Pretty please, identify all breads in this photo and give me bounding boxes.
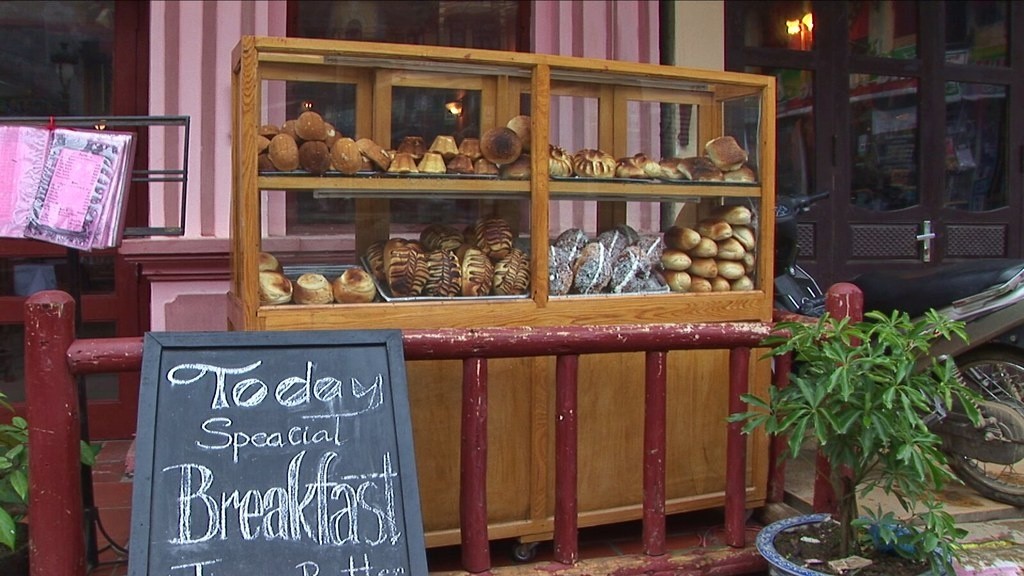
[548,136,756,183]
[257,111,531,173]
[258,214,532,304]
[548,204,756,294]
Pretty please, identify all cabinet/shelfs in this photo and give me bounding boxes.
[227,34,777,566]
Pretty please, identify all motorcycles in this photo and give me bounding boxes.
[750,189,1024,512]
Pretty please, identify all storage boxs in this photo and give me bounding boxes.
[746,17,1008,119]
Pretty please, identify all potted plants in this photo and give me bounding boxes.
[0,390,104,576]
[721,308,984,576]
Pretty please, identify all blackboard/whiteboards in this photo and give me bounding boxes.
[128,328,431,576]
